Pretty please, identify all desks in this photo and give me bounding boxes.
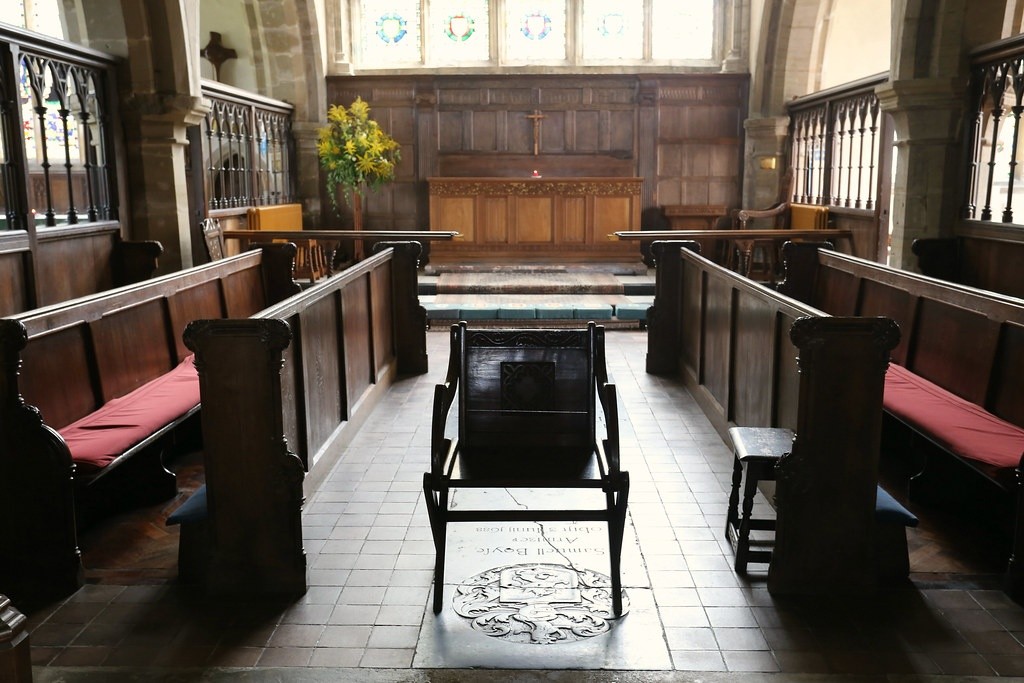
[426,176,646,264]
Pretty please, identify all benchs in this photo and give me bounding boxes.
[772,234,1024,607]
[0,238,303,595]
[643,236,921,608]
[159,239,431,606]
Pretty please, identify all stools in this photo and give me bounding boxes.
[723,418,802,578]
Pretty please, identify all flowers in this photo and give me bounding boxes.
[311,90,406,217]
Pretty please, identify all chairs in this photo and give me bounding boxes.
[418,308,634,626]
[159,310,312,615]
